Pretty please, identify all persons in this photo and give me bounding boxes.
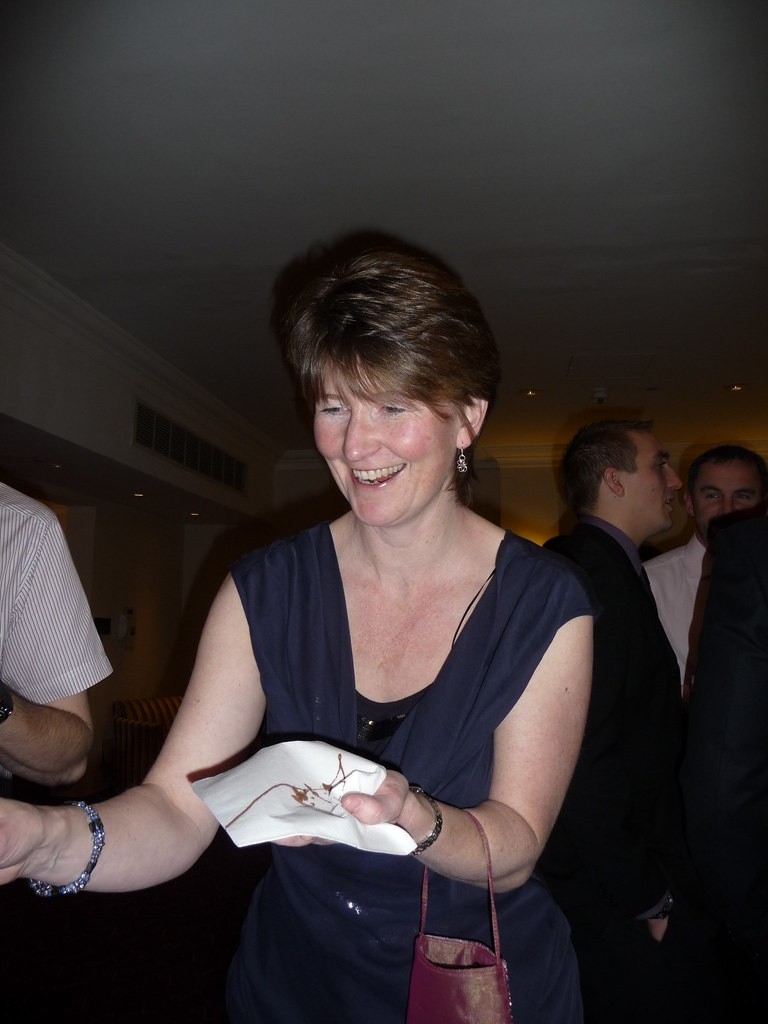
[685,496,765,1024]
[0,479,113,1019]
[638,442,765,700]
[0,245,599,1024]
[541,416,690,1022]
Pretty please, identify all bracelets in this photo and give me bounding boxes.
[650,891,674,920]
[0,680,14,725]
[28,798,107,899]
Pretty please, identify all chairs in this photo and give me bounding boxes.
[112,695,184,792]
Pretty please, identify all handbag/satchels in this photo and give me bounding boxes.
[406,804,515,1024]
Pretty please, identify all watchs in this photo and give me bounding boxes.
[399,784,444,861]
